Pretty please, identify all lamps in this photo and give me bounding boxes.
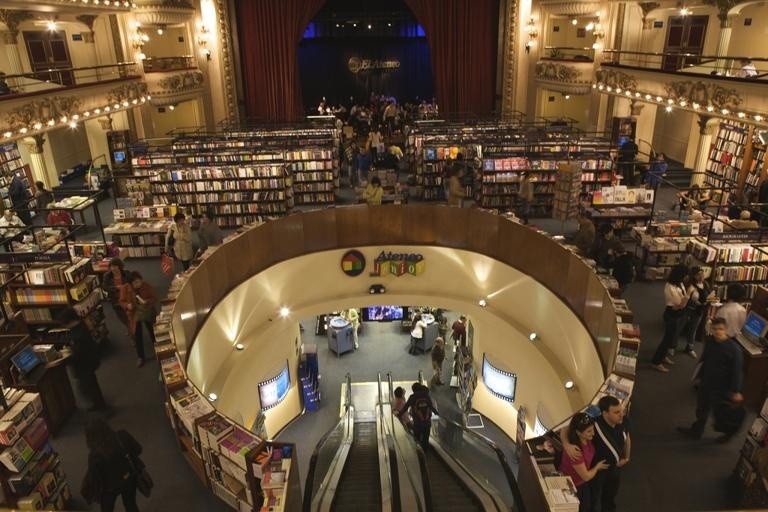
[127,20,149,64]
[196,23,216,62]
[526,12,540,55]
[590,10,607,51]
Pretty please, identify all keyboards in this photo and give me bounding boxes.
[736,335,762,355]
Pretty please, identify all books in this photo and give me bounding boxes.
[0,125,765,511]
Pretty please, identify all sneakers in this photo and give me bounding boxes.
[137,358,142,367]
[651,349,696,372]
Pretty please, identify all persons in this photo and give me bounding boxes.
[318,89,440,124]
[730,55,758,80]
[0,72,14,94]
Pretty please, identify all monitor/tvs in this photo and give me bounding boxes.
[742,310,768,338]
[11,344,40,376]
[360,306,409,322]
[618,137,629,145]
[114,152,125,161]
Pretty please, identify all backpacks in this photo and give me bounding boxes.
[414,395,431,421]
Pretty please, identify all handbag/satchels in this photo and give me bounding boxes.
[714,401,744,432]
[168,230,174,245]
[134,305,153,322]
[162,254,173,273]
[136,469,153,497]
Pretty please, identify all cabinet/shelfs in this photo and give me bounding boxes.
[327,317,358,356]
[116,123,338,260]
[154,346,296,511]
[410,320,440,356]
[361,118,518,235]
